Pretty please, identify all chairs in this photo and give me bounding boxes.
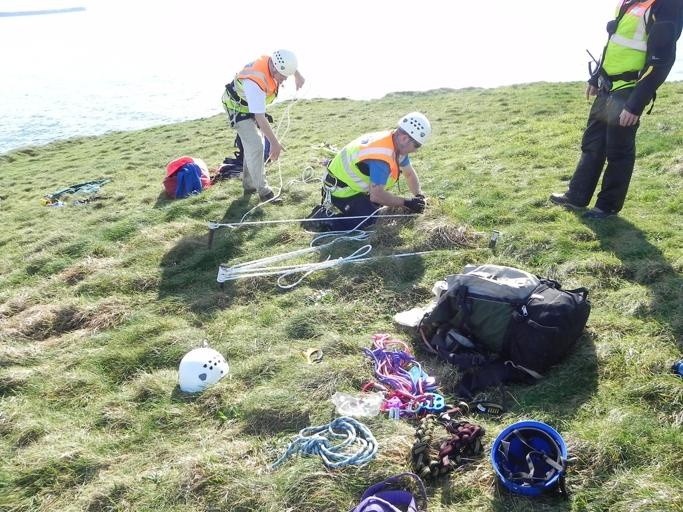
[301,205,331,230]
[243,188,283,205]
[549,192,618,219]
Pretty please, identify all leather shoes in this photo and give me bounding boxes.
[405,196,426,214]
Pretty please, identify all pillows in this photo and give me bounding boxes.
[491,420,568,495]
[178,346,228,393]
[270,49,297,78]
[398,112,430,145]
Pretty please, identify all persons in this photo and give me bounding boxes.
[300,111,433,233]
[222,50,303,203]
[548,0,683,223]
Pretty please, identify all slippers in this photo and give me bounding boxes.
[162,156,211,199]
[420,264,589,381]
